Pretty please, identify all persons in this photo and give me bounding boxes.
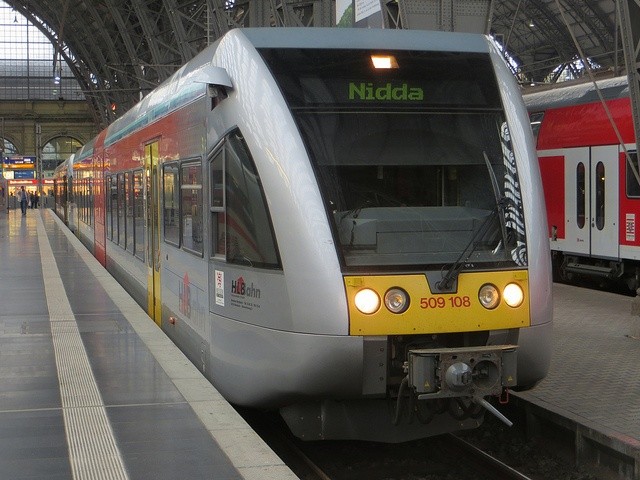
[18,186,28,216]
[28,190,39,209]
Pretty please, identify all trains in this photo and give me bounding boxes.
[517,72,640,296]
[53,26,555,445]
